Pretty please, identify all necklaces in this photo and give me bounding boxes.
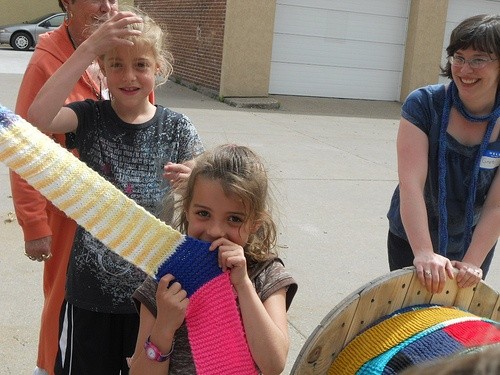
[71,37,94,64]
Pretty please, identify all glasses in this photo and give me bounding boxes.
[447,54,498,69]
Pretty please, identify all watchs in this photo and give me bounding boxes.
[144,335,175,362]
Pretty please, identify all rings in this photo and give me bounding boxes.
[24,253,50,261]
[475,270,480,274]
[425,271,431,276]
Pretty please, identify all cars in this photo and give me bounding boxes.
[0,12,67,50]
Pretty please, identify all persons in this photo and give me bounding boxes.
[386,14,500,294]
[9,0,154,375]
[129,144,298,375]
[26,5,206,375]
